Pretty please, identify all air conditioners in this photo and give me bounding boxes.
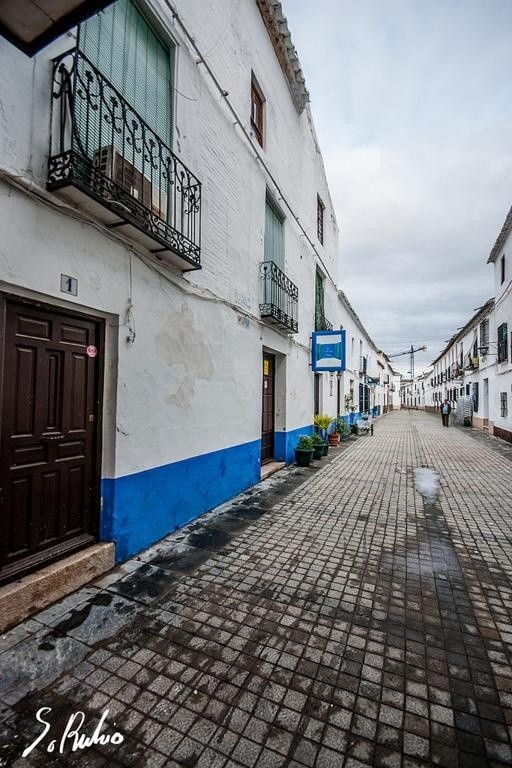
[88,145,152,221]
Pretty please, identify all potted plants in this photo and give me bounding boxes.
[293,414,353,466]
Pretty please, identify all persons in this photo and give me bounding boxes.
[439,399,451,427]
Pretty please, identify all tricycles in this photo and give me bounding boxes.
[353,410,373,436]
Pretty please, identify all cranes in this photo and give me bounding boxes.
[386,344,428,378]
